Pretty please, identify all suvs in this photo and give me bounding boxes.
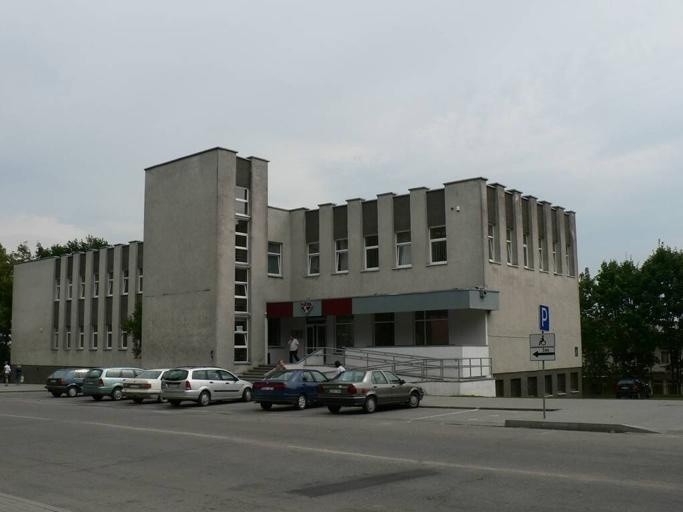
[158,365,254,408]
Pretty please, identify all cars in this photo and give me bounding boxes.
[250,368,330,412]
[120,367,172,404]
[44,366,89,398]
[615,378,651,399]
[317,367,425,414]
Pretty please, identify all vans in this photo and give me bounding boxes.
[79,367,146,401]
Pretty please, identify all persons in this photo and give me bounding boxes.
[13,363,22,385]
[1,360,12,387]
[335,360,346,376]
[276,360,287,371]
[287,334,300,363]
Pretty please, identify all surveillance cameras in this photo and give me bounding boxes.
[456,205,460,212]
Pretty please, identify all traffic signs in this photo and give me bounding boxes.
[528,332,556,362]
